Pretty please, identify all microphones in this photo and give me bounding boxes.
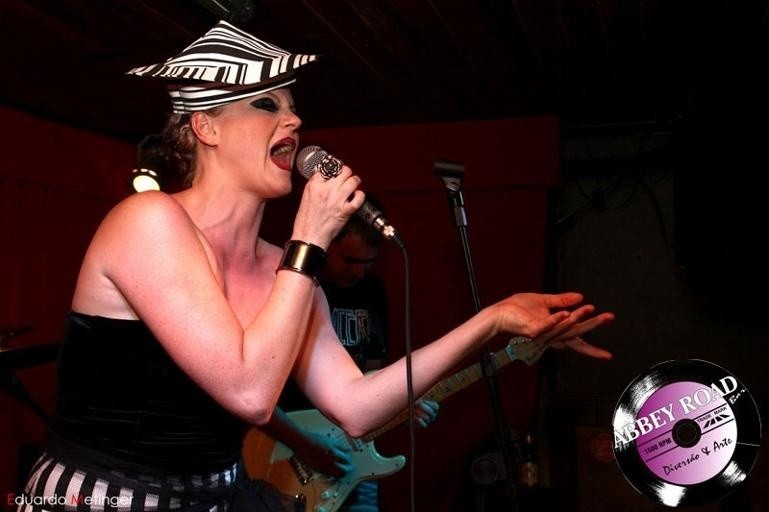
[296,145,400,241]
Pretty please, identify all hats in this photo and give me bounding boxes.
[125,19,318,115]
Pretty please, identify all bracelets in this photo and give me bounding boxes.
[276,240,327,288]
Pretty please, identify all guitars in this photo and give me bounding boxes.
[242,332,548,511]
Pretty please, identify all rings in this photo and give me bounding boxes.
[317,155,343,179]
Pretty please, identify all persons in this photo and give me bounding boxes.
[14,19,614,512]
[239,192,440,512]
